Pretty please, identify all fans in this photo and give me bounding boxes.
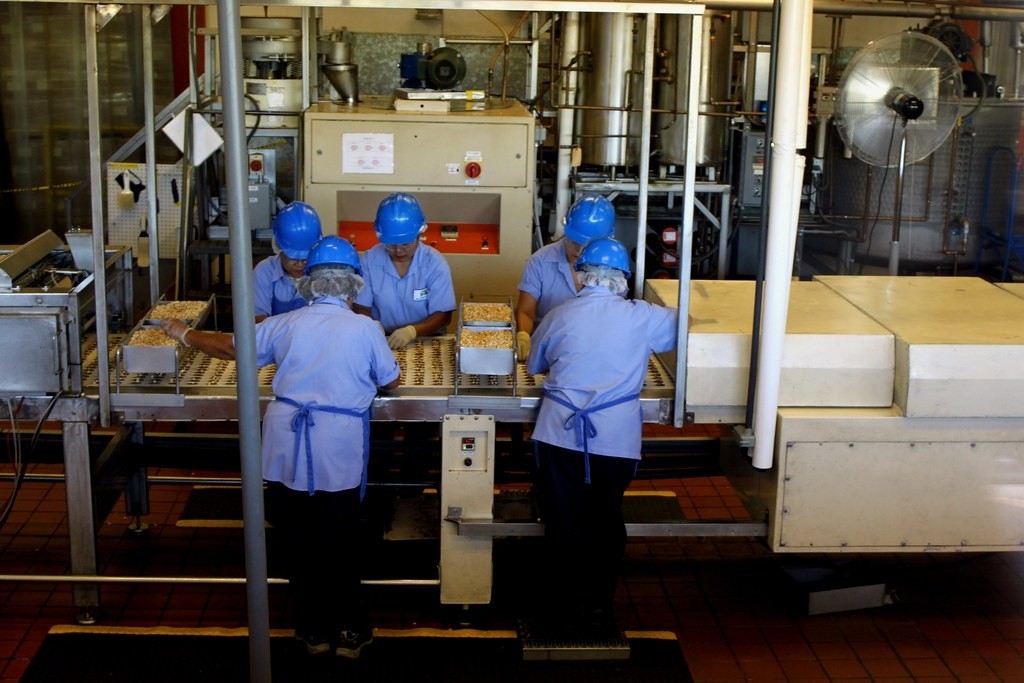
[833,29,966,276]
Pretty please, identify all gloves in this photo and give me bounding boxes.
[389,325,417,350]
[516,331,532,362]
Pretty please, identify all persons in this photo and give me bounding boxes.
[159,235,401,658]
[517,193,615,361]
[526,236,692,627]
[253,201,322,322]
[353,193,457,483]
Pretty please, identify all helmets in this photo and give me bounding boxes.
[574,238,632,278]
[374,192,426,246]
[306,235,363,270]
[273,201,323,260]
[565,193,617,246]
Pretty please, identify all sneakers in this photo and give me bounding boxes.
[300,634,331,656]
[337,627,374,660]
